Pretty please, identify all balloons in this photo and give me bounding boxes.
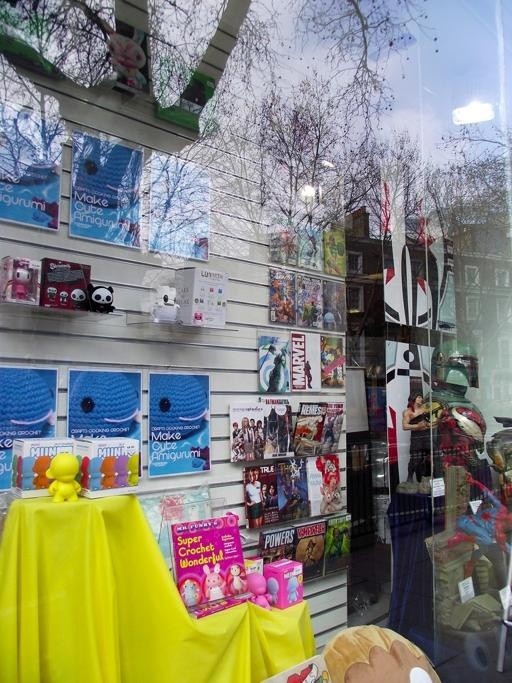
[4,267,112,311]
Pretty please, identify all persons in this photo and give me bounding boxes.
[267,345,287,392]
[305,360,313,389]
[448,473,512,578]
[11,454,138,503]
[394,391,448,494]
[233,411,347,578]
[327,234,341,275]
